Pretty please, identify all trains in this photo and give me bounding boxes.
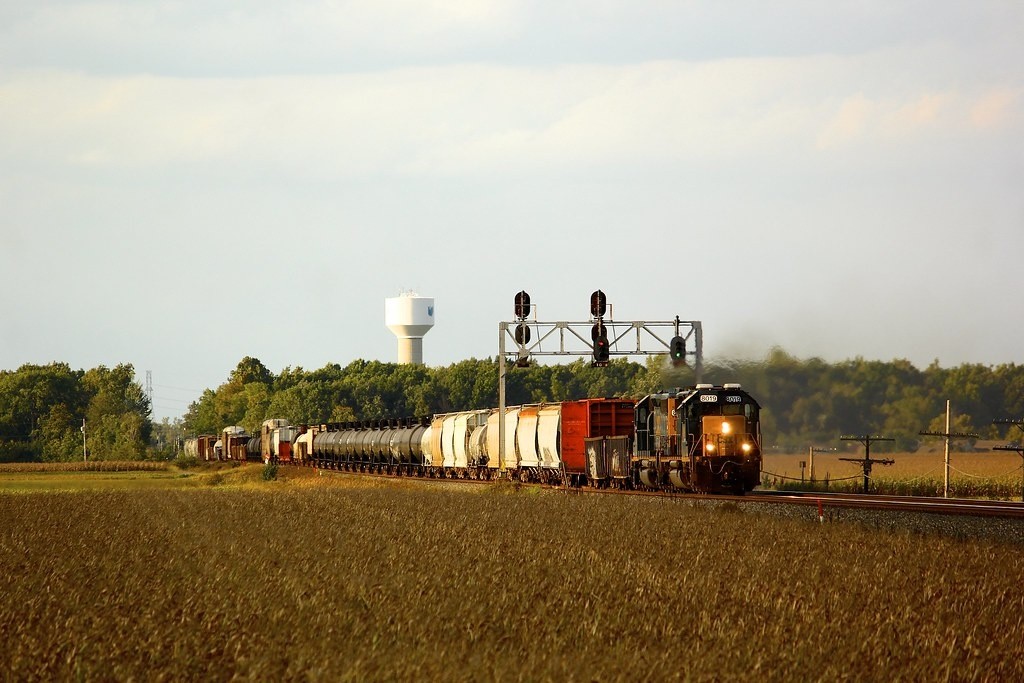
[182,381,762,498]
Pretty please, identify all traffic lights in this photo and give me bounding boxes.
[675,340,683,359]
[594,336,609,361]
